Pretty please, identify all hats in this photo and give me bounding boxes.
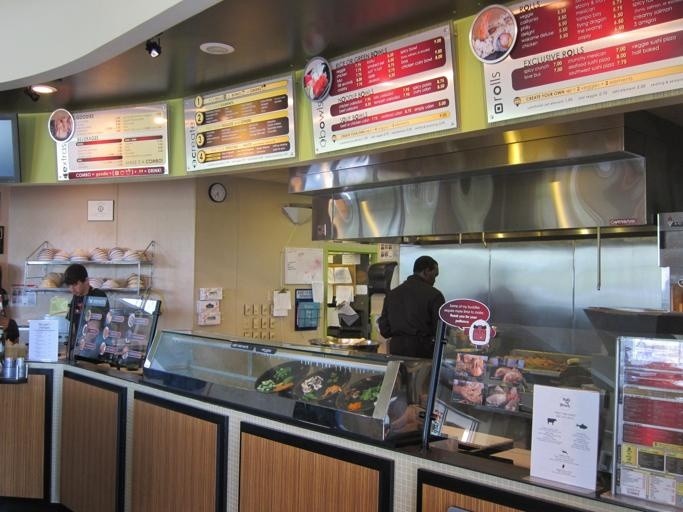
[59,265,88,287]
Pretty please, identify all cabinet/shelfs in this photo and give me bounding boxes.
[24,240,155,295]
[145,330,413,442]
[424,319,682,512]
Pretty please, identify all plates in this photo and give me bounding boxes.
[27,244,150,292]
[251,357,382,413]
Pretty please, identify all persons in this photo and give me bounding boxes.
[376,256,445,360]
[62,264,110,365]
[0,288,19,367]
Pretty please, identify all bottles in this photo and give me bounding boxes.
[0,326,27,381]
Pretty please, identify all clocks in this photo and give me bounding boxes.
[208,183,227,202]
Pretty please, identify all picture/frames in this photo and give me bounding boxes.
[468,4,518,64]
[47,108,75,144]
[302,57,333,102]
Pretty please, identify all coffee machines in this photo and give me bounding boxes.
[655,210,683,314]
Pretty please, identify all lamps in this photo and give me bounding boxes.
[145,38,161,57]
[25,85,57,101]
